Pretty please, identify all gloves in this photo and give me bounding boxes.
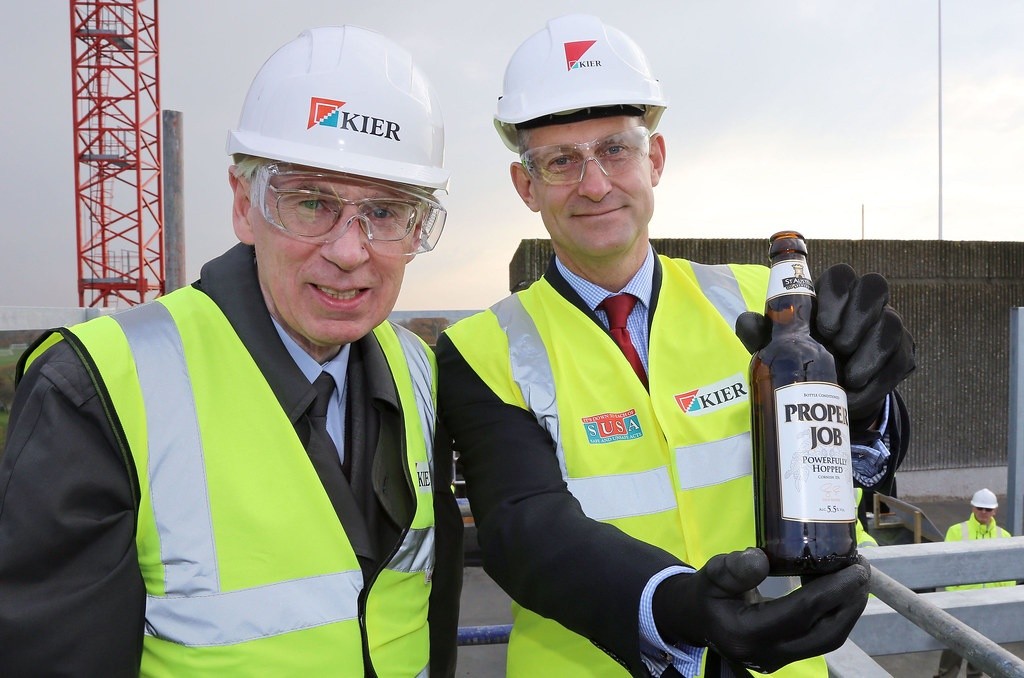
[734,263,917,437]
[651,546,872,674]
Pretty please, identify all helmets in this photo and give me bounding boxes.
[491,14,667,157]
[225,25,450,195]
[970,488,998,510]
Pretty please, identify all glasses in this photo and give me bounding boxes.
[247,157,446,258]
[518,127,651,184]
[976,506,992,512]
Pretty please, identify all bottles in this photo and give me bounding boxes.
[746,230,860,575]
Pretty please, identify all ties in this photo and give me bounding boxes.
[594,292,650,396]
[308,372,342,471]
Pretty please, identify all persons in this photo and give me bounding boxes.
[0,26,464,678]
[853,486,879,599]
[933,488,1016,678]
[434,14,916,678]
